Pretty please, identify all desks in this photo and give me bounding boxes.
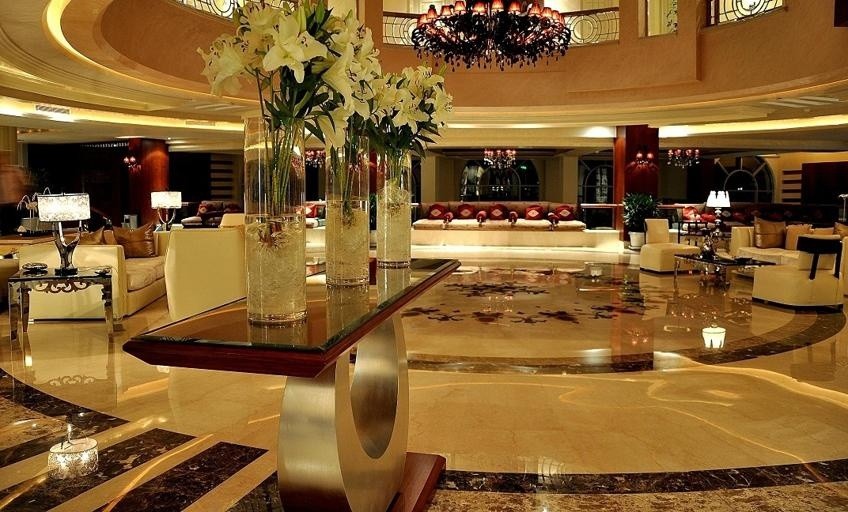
[122,259,462,512]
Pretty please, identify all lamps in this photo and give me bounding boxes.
[47,412,98,478]
[706,190,730,236]
[150,191,182,232]
[124,155,138,168]
[664,148,702,169]
[411,0,571,73]
[482,149,517,170]
[636,151,654,166]
[700,306,726,351]
[36,192,93,276]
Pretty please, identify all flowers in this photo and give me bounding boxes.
[313,18,375,228]
[196,1,353,248]
[367,61,454,219]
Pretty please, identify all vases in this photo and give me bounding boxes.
[243,115,308,327]
[324,133,369,286]
[375,147,411,270]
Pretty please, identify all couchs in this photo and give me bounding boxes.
[181,198,241,228]
[18,221,172,322]
[751,236,843,310]
[164,217,325,323]
[411,201,597,247]
[730,217,848,295]
[639,218,699,273]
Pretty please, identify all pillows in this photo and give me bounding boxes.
[555,205,576,222]
[524,204,545,221]
[197,202,212,217]
[682,206,698,221]
[78,225,107,245]
[832,221,848,238]
[488,204,508,221]
[428,204,445,220]
[753,216,786,250]
[305,205,316,218]
[111,222,156,258]
[455,204,475,220]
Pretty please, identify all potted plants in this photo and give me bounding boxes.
[621,192,664,250]
